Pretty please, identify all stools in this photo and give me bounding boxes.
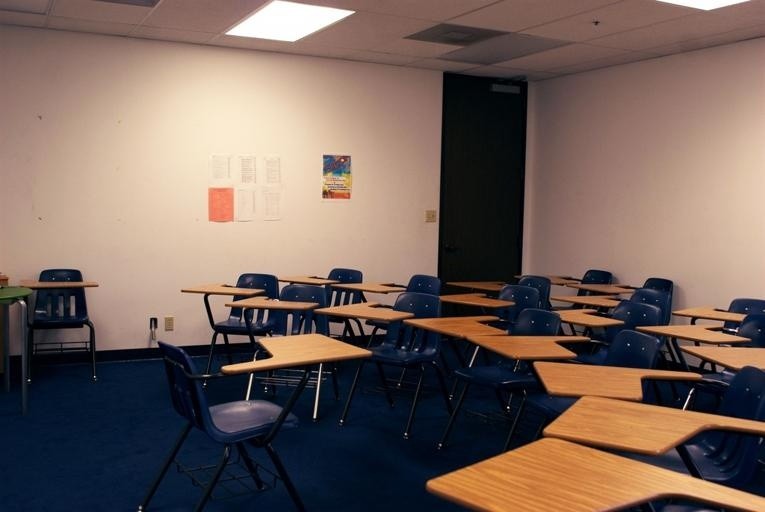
[0,287,33,417]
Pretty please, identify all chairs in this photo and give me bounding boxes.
[181,274,279,389]
[331,275,441,295]
[313,292,452,440]
[224,285,340,420]
[439,285,540,324]
[636,312,765,373]
[402,307,561,453]
[281,268,365,342]
[672,298,765,346]
[463,329,661,453]
[514,270,612,311]
[552,299,661,364]
[533,361,765,486]
[446,276,551,308]
[567,277,673,295]
[20,268,99,385]
[138,333,373,512]
[551,287,672,325]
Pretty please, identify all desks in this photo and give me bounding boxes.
[680,345,765,371]
[425,437,765,512]
[543,396,765,497]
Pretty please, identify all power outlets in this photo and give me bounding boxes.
[165,317,173,331]
